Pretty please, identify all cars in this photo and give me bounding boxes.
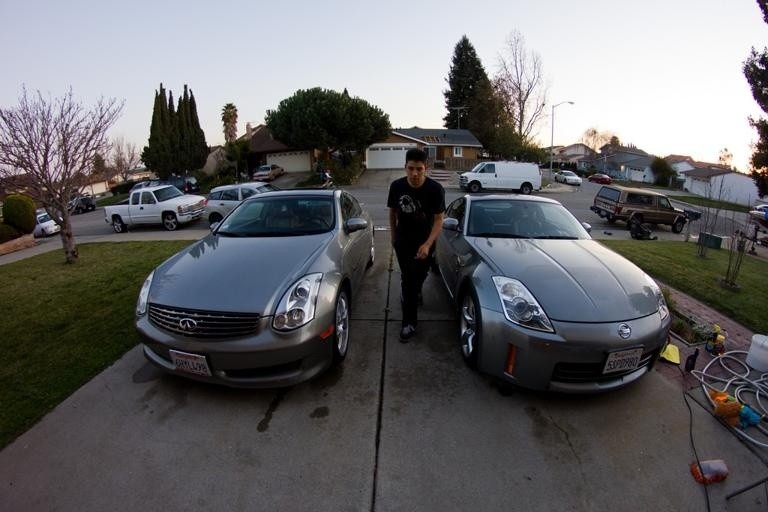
[204,182,278,224]
[34,212,63,237]
[747,202,768,220]
[129,176,200,194]
[588,173,612,185]
[254,163,284,181]
[66,197,96,214]
[432,194,670,393]
[132,190,377,388]
[552,169,583,186]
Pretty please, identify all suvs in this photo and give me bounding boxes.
[590,184,686,232]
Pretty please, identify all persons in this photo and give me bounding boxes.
[385,149,447,343]
[320,168,335,190]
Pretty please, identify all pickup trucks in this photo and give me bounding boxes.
[102,186,204,233]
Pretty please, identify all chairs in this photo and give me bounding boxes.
[265,204,333,228]
[463,204,544,234]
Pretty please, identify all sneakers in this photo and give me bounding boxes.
[399,321,418,339]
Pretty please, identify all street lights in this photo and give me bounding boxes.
[550,101,576,184]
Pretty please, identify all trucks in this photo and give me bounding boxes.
[459,161,542,193]
